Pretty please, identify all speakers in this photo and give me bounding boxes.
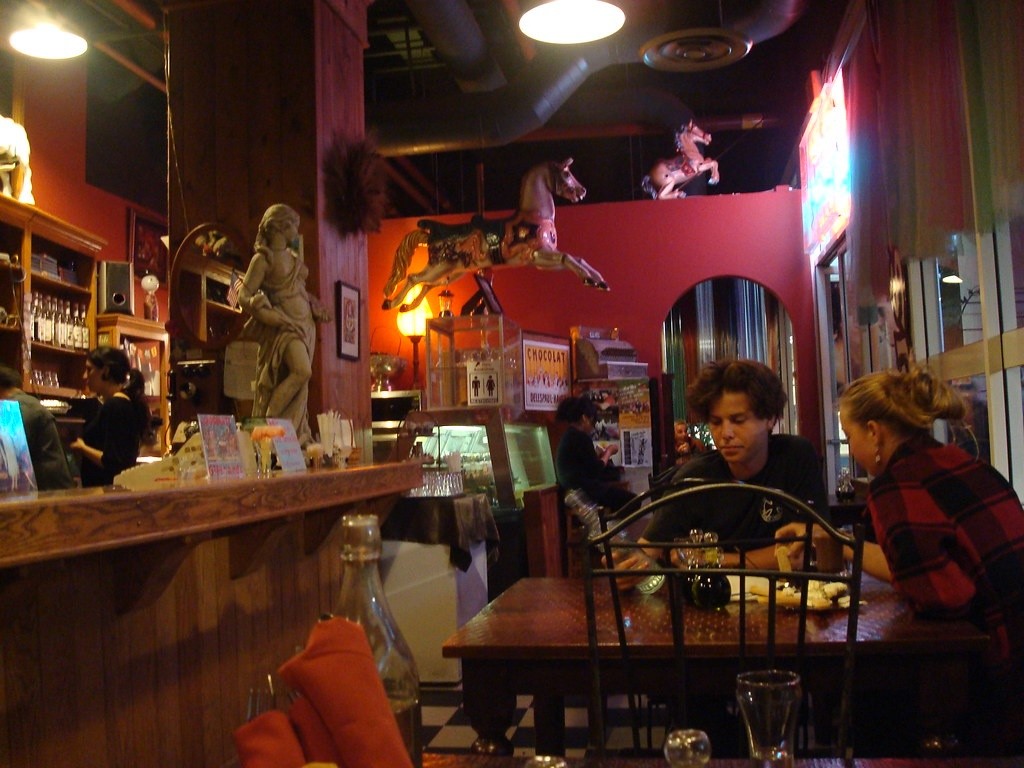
[97,260,134,316]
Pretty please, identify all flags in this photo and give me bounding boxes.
[226,270,244,309]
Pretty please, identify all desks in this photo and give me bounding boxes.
[0,458,427,768]
[421,516,1024,768]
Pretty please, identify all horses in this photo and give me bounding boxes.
[640,118,720,200]
[382,150,610,312]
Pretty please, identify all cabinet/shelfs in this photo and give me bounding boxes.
[0,192,172,488]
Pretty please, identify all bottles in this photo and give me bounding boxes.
[317,515,422,768]
[565,490,664,593]
[675,530,732,608]
[17,290,91,350]
[29,370,61,389]
[834,467,856,499]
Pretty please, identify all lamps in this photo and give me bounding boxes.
[9,5,88,59]
[939,266,963,283]
[397,282,433,391]
[516,0,626,45]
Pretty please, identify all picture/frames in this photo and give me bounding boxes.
[460,273,504,314]
[336,279,360,361]
[128,207,170,289]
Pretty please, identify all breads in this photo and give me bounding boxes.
[748,547,848,609]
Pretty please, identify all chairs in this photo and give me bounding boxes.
[566,467,866,760]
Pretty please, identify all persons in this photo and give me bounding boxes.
[236,202,335,450]
[1,366,72,491]
[772,364,1024,759]
[553,395,640,565]
[616,357,828,570]
[671,419,706,466]
[66,346,154,488]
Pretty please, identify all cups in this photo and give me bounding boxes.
[815,525,854,578]
[403,471,467,498]
[735,669,802,768]
